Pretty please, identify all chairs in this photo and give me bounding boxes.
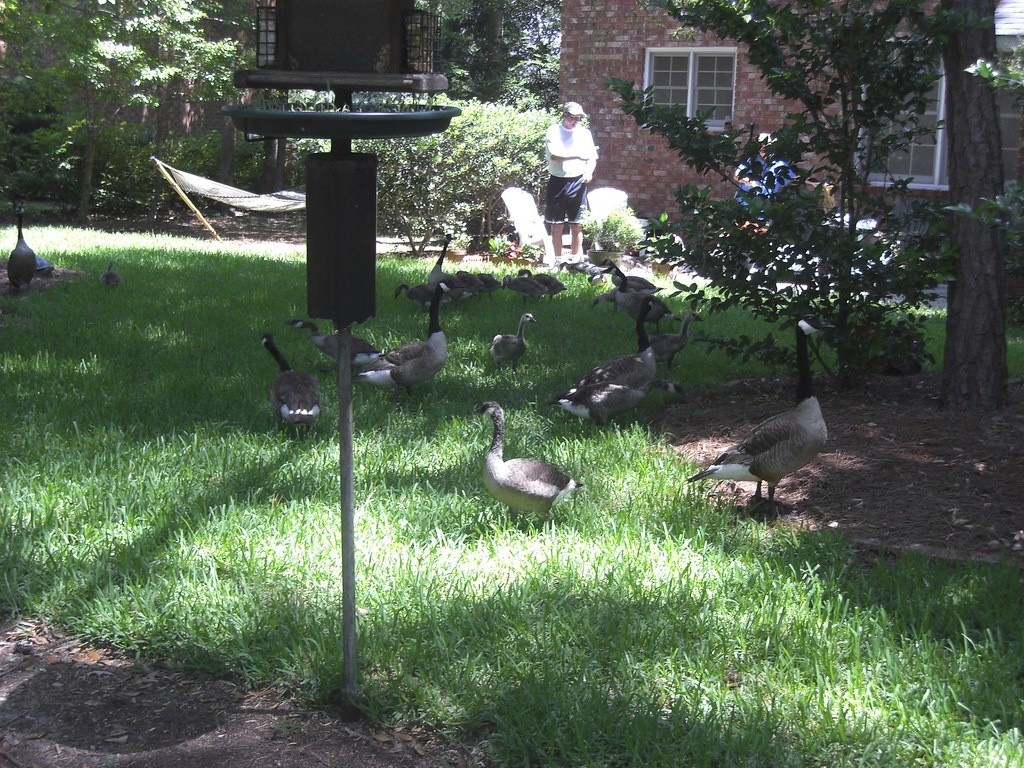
[501,189,574,262]
[587,187,648,246]
[880,199,933,271]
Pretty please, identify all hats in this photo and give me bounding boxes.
[757,133,778,144]
[563,102,589,118]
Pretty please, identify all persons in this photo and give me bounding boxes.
[732,132,821,236]
[544,101,598,272]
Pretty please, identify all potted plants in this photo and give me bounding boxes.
[487,235,514,265]
[445,237,473,263]
[640,212,680,278]
[514,246,545,268]
[580,206,640,266]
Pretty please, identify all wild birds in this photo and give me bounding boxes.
[561,258,681,336]
[648,312,703,370]
[490,314,537,374]
[561,378,685,426]
[100,262,121,286]
[352,277,466,399]
[564,295,664,391]
[687,314,835,513]
[394,234,501,316]
[503,274,548,306]
[473,401,585,530]
[261,333,322,435]
[36,254,55,275]
[285,319,383,377]
[517,268,567,300]
[7,205,36,291]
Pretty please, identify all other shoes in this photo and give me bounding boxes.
[553,260,562,272]
